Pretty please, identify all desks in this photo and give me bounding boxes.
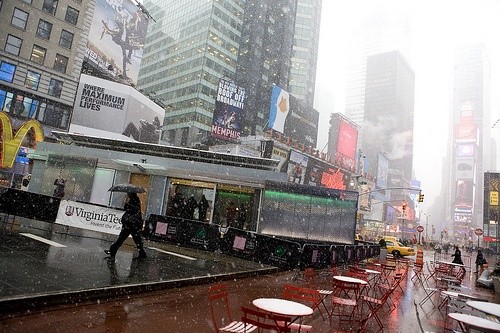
[332,275,368,289]
[442,290,500,333]
[438,260,466,268]
[359,268,381,276]
[252,298,313,329]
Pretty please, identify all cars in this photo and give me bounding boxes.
[384,239,416,258]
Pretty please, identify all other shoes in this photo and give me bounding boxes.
[104,249,115,255]
[479,273,481,276]
[132,255,148,260]
[474,271,478,273]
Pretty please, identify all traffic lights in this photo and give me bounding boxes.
[419,194,424,202]
[403,204,406,210]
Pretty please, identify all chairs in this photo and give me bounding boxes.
[208,257,462,333]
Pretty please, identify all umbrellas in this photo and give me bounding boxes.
[110,184,145,194]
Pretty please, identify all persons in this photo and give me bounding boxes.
[293,163,303,183]
[105,192,146,258]
[364,236,494,274]
[53,178,64,199]
[170,192,245,229]
